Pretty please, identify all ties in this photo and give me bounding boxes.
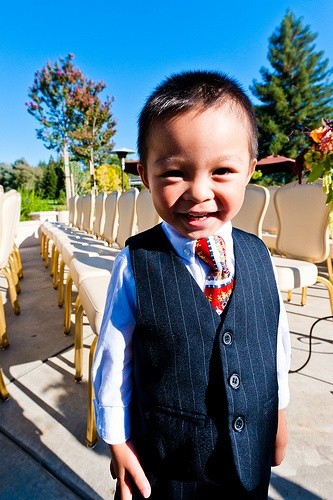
[195,236,236,321]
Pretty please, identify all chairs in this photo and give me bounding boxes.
[0,177,333,449]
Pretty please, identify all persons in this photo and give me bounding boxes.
[91,70,291,500]
